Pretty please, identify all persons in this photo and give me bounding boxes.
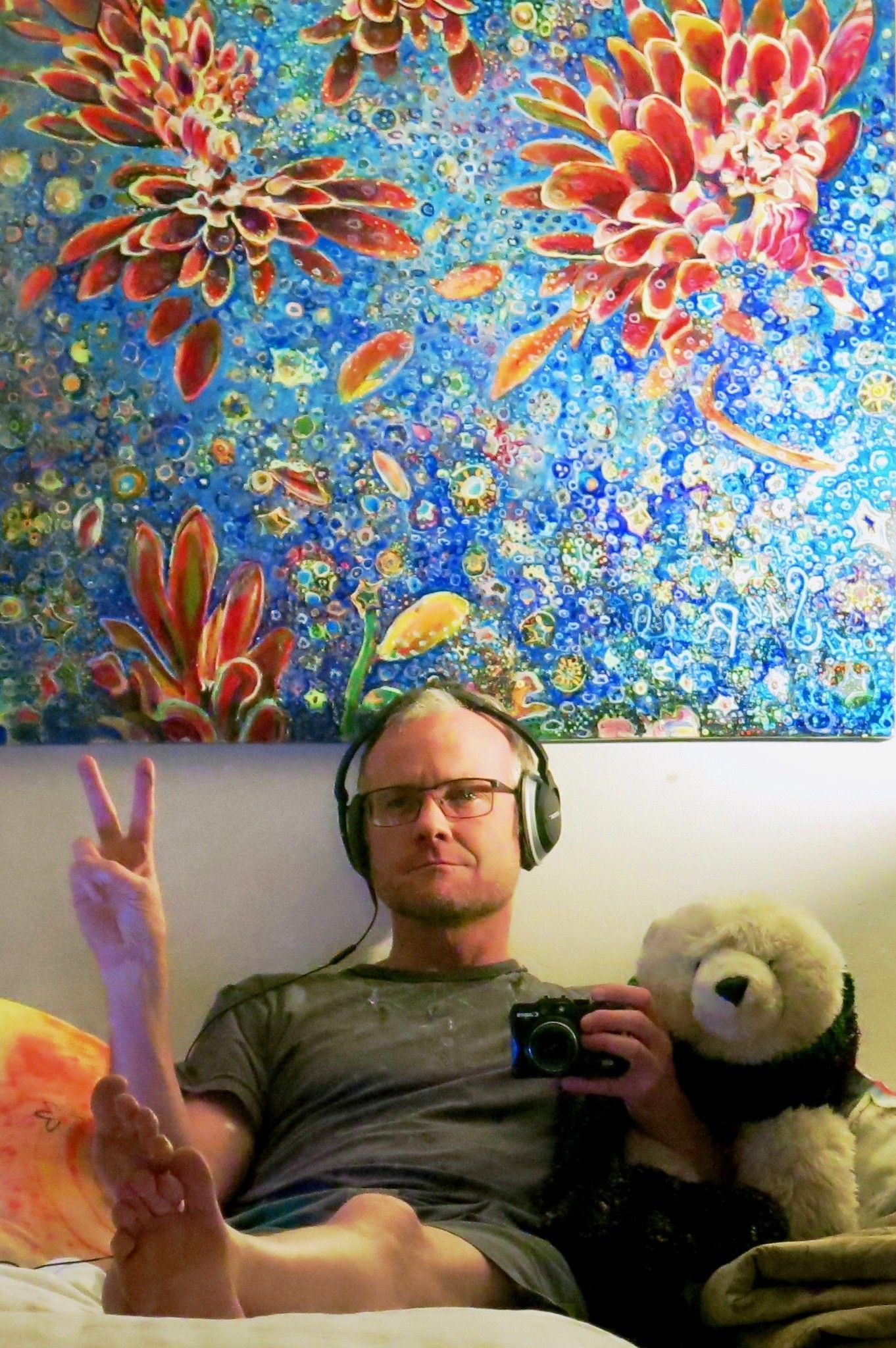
[66,686,706,1317]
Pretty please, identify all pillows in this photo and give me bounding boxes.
[0,997,112,1269]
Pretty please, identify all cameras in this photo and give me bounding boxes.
[508,997,631,1078]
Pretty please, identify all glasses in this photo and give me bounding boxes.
[358,777,518,828]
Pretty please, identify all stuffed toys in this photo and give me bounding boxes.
[632,891,896,1246]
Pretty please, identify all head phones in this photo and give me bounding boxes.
[334,689,561,882]
